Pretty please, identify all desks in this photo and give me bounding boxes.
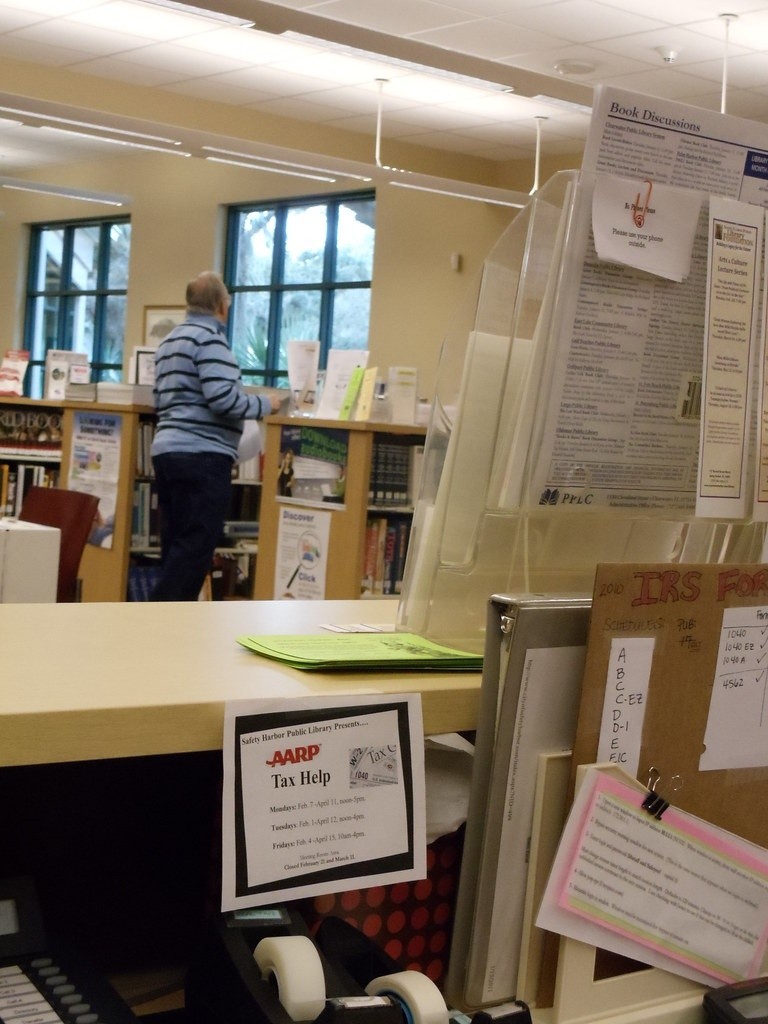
[0,597,493,767]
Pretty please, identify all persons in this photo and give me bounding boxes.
[149,273,280,602]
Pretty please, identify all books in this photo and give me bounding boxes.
[0,423,264,600]
[361,441,445,595]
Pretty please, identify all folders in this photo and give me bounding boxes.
[447,589,594,1012]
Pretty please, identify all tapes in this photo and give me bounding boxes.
[364,969,450,1024]
[253,935,327,1021]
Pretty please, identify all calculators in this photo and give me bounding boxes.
[703,977,768,1024]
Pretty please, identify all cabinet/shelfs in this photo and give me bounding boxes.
[0,396,448,602]
